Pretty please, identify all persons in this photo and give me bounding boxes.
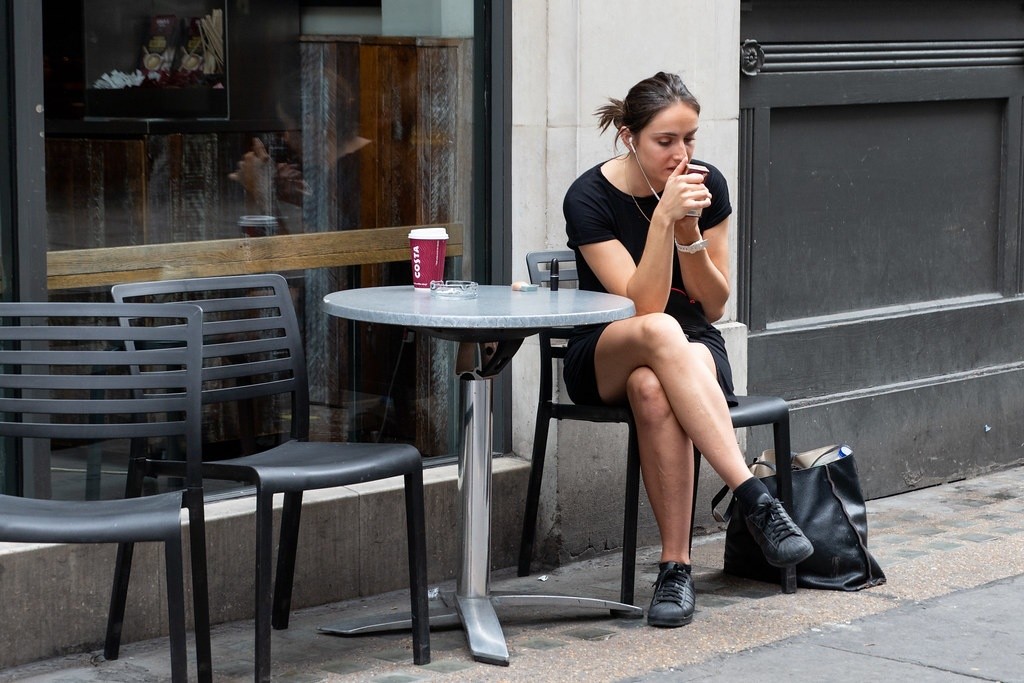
[563,71,816,628]
[226,65,375,361]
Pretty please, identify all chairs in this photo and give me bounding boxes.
[517,249,796,619]
[1,274,431,683]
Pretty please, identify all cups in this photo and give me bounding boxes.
[408,228,450,290]
[238,216,277,237]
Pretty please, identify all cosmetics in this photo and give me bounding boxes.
[512,282,538,292]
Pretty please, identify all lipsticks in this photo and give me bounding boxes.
[550,257,560,291]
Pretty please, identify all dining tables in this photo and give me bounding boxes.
[318,284,644,666]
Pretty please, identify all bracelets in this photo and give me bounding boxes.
[674,236,708,254]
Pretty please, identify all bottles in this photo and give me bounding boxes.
[813,447,852,467]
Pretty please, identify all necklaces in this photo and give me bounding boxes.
[625,152,651,223]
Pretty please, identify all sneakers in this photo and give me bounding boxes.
[745,492,814,568]
[646,560,695,629]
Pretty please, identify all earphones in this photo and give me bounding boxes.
[629,137,637,153]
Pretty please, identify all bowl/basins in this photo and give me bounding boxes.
[140,87,225,118]
[91,87,149,114]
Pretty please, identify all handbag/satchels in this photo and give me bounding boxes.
[710,443,887,592]
